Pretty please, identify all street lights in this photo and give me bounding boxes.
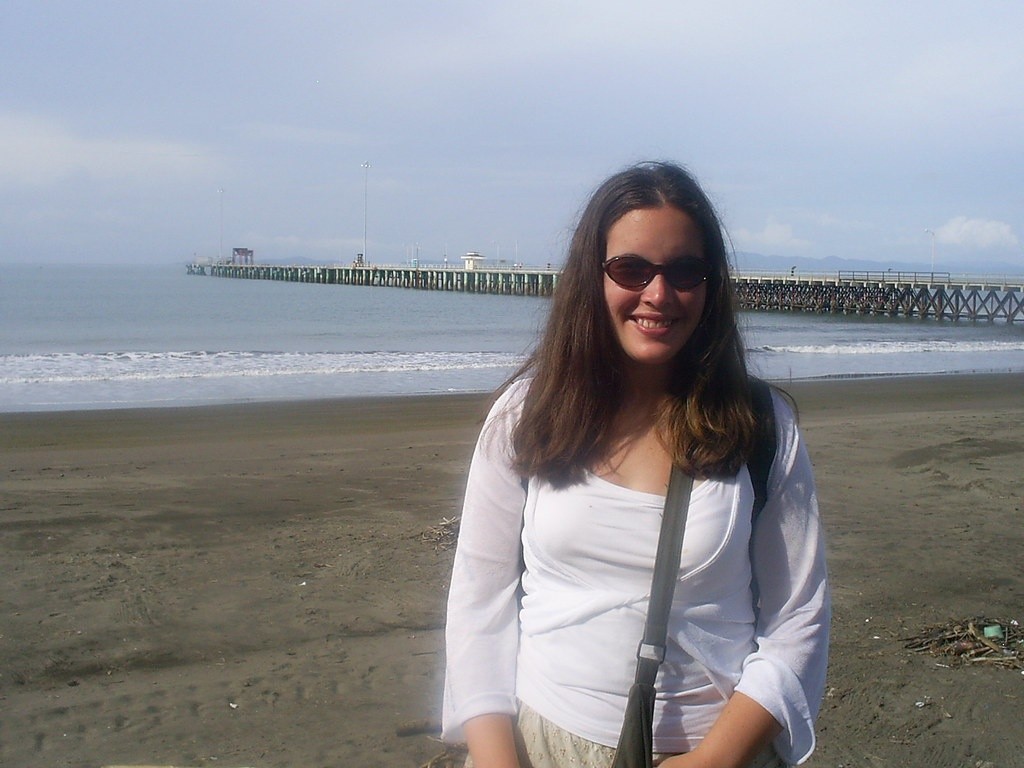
[218,187,226,266]
[359,160,375,267]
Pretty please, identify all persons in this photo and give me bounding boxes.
[440,158,829,767]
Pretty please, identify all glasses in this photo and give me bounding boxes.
[601,254,714,290]
